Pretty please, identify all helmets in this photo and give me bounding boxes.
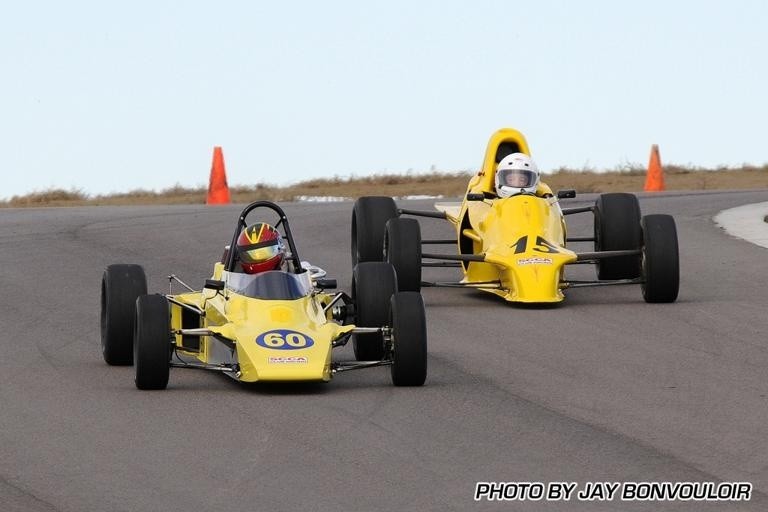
[235,223,286,273]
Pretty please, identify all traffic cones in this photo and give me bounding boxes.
[203,146,233,207]
[642,145,667,193]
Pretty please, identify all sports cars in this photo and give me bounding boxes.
[99,200,430,394]
[350,129,680,307]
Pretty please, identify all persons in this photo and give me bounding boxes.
[237,223,295,272]
